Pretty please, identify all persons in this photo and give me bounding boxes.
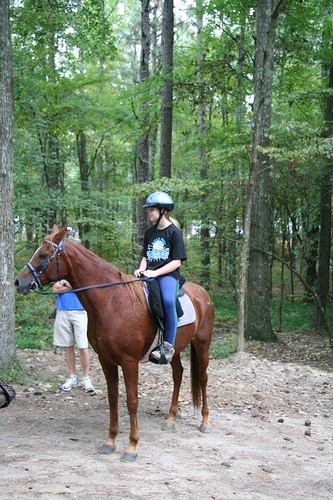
[134,191,186,365]
[52,279,95,394]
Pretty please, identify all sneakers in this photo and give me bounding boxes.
[60,377,77,390]
[80,378,94,392]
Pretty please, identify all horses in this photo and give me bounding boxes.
[14,225,215,464]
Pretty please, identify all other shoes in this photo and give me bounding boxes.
[151,341,174,364]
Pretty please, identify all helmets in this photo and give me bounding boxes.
[142,192,173,211]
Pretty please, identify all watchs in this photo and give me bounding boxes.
[63,280,67,286]
